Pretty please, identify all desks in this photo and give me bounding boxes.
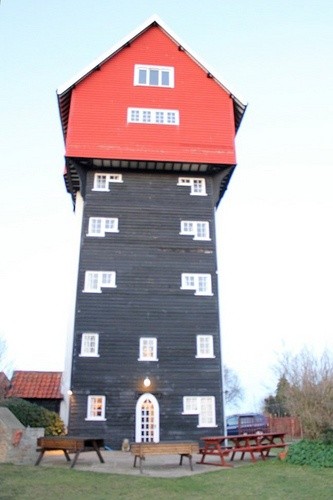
[202,432,287,466]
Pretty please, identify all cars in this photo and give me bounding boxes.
[225,413,270,439]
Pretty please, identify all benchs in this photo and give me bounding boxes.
[220,442,289,467]
[197,443,272,464]
